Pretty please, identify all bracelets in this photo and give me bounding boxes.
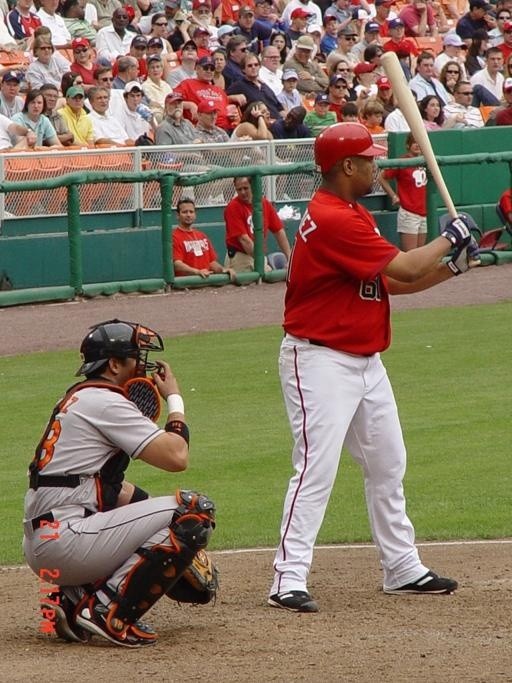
[164,392,184,420]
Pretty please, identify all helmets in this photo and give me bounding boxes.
[314,122,386,173]
[74,319,164,387]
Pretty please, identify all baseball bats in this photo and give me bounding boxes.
[380,50,484,268]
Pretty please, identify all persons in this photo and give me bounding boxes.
[494,160,511,243]
[0,0,512,213]
[377,129,435,254]
[21,317,220,651]
[218,172,293,278]
[266,121,482,618]
[166,197,238,282]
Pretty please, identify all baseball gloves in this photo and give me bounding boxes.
[164,550,220,605]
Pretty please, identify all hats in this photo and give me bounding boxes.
[0,0,240,114]
[240,0,512,104]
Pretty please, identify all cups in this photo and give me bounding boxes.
[135,103,154,122]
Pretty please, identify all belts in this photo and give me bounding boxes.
[32,507,96,530]
[284,331,327,347]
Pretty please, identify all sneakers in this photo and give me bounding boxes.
[76,594,158,648]
[383,572,457,593]
[268,590,318,611]
[40,590,88,643]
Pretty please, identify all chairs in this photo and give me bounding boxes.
[2,140,184,215]
[380,36,446,56]
[1,47,98,72]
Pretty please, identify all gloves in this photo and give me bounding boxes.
[441,217,471,248]
[446,234,481,276]
